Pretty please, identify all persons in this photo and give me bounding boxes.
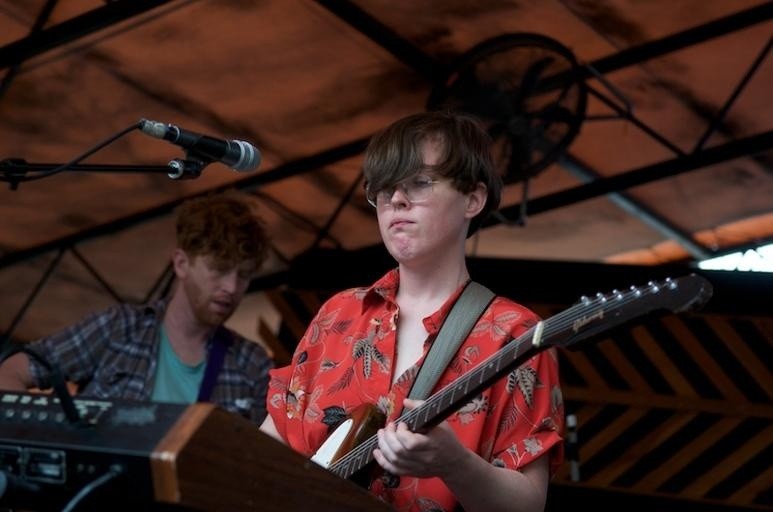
[1,193,277,431]
[260,108,568,510]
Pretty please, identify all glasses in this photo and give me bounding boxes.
[363,174,450,208]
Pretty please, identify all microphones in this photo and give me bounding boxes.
[137,118,261,172]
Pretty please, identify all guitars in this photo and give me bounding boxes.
[307,271,713,484]
